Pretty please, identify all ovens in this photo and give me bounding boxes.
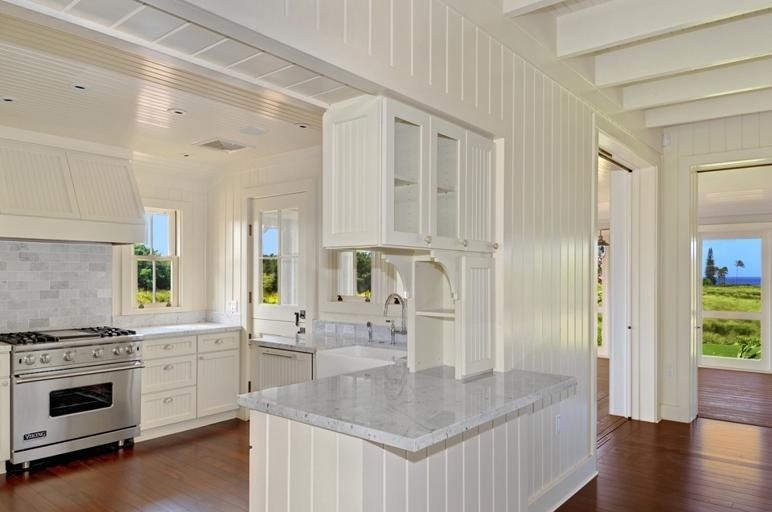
[10,341,146,464]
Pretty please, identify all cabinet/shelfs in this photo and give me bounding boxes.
[406,251,494,381]
[321,94,497,253]
[127,331,240,443]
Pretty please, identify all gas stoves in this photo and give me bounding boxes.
[0,325,144,352]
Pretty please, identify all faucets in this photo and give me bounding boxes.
[383,291,406,333]
